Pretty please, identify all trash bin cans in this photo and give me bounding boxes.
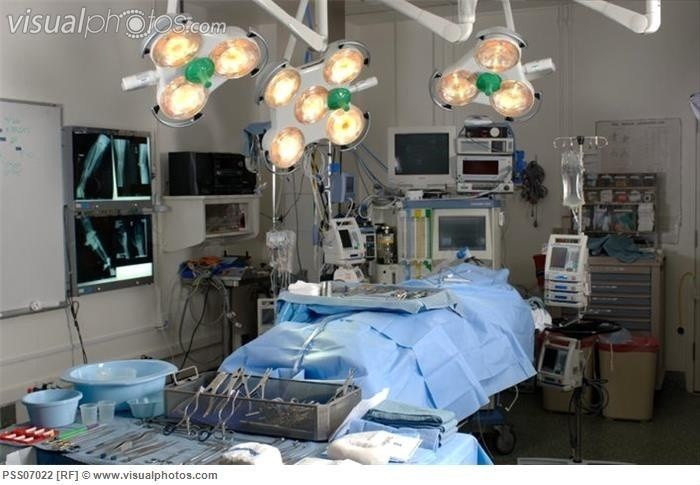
[537,330,596,415]
[596,336,659,424]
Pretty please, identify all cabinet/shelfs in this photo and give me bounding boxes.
[563,252,665,392]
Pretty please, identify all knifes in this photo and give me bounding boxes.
[42,419,309,465]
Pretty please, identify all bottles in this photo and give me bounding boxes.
[322,279,333,296]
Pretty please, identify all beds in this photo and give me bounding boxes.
[221,260,534,445]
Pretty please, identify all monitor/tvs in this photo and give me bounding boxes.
[64,205,157,298]
[430,209,492,260]
[387,125,457,191]
[63,126,155,206]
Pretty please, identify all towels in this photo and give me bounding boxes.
[365,401,462,446]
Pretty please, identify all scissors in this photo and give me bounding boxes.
[163,366,357,444]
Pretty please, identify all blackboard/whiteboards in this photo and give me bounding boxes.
[0,98,72,320]
[595,117,682,244]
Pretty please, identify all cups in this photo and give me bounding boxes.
[95,366,137,380]
[97,398,117,422]
[80,400,100,426]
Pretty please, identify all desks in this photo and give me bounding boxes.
[180,269,276,370]
[1,400,483,464]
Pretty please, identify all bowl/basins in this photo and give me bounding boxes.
[21,388,86,431]
[60,357,178,413]
[125,397,161,418]
